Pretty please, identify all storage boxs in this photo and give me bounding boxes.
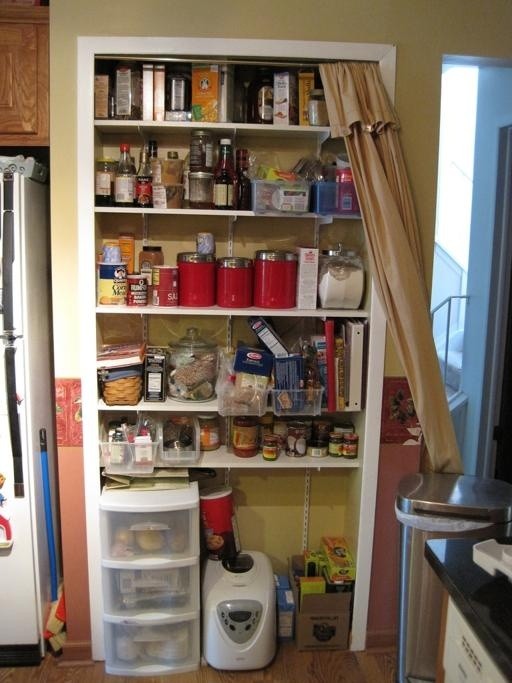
[288,555,351,648]
[272,388,325,417]
[155,415,201,463]
[252,180,311,213]
[312,183,361,216]
[100,415,159,475]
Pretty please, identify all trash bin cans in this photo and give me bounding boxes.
[394,471,512,683]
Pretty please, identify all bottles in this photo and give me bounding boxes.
[318,244,365,309]
[108,422,116,441]
[113,145,190,207]
[254,66,273,124]
[309,90,329,125]
[110,432,125,465]
[166,328,218,404]
[139,245,164,273]
[211,139,251,209]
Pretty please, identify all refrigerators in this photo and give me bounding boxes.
[1,168,59,665]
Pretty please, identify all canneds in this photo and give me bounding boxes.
[188,172,211,207]
[137,174,152,207]
[229,415,357,461]
[198,412,220,450]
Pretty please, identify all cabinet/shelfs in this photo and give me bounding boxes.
[96,482,200,676]
[1,1,49,146]
[77,39,397,653]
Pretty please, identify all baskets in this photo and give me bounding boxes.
[103,376,143,406]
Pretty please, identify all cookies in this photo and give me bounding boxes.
[205,536,226,551]
